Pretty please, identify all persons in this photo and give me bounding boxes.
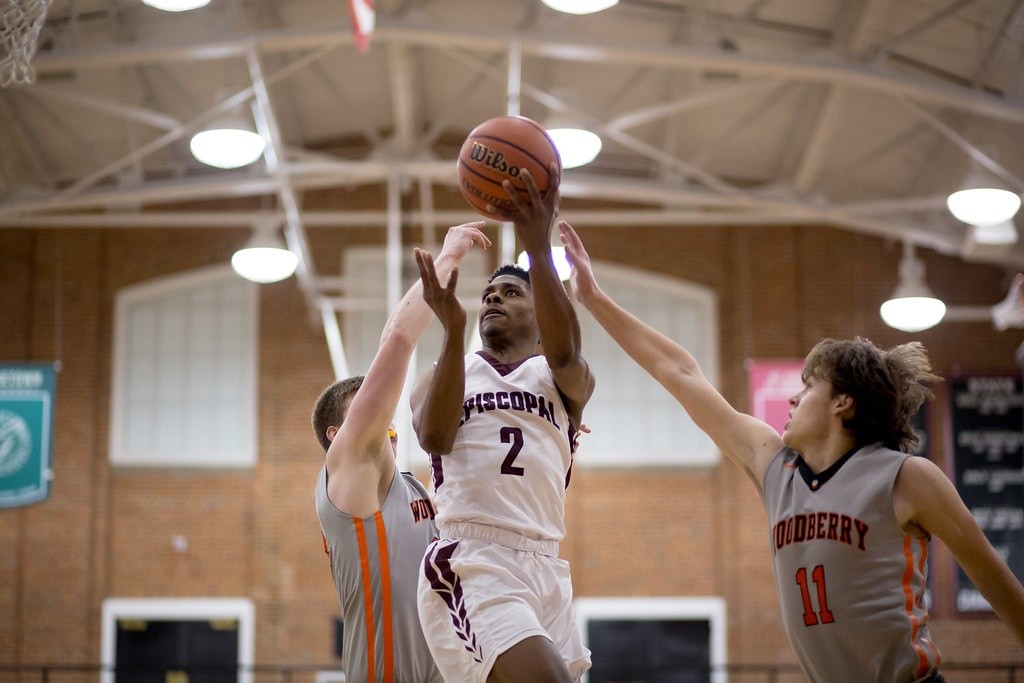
[558,221,1024,683]
[409,161,596,683]
[311,220,492,683]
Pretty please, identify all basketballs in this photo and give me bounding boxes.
[457,115,563,222]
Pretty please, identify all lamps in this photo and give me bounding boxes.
[190,85,266,170]
[230,204,299,284]
[879,242,946,333]
[947,138,1021,227]
